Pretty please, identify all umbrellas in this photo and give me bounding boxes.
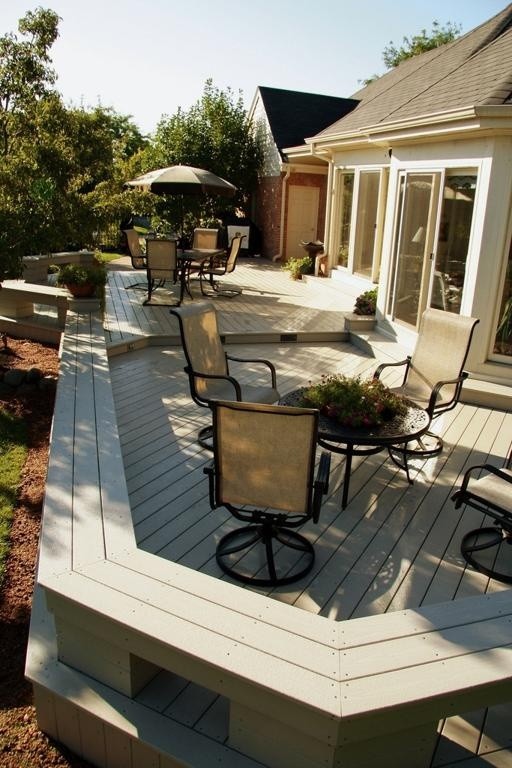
[400,180,471,217]
[124,163,237,254]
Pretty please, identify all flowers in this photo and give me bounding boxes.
[294,372,405,426]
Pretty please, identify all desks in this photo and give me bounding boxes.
[274,388,430,508]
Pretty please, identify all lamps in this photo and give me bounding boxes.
[409,225,427,255]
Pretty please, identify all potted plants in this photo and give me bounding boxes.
[281,255,312,281]
[58,263,107,299]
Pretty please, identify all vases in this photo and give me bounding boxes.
[325,403,383,434]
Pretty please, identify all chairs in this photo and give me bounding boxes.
[371,307,480,454]
[451,442,511,583]
[124,225,245,309]
[171,301,281,454]
[203,400,330,589]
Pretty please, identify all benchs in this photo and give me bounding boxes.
[21,249,97,285]
